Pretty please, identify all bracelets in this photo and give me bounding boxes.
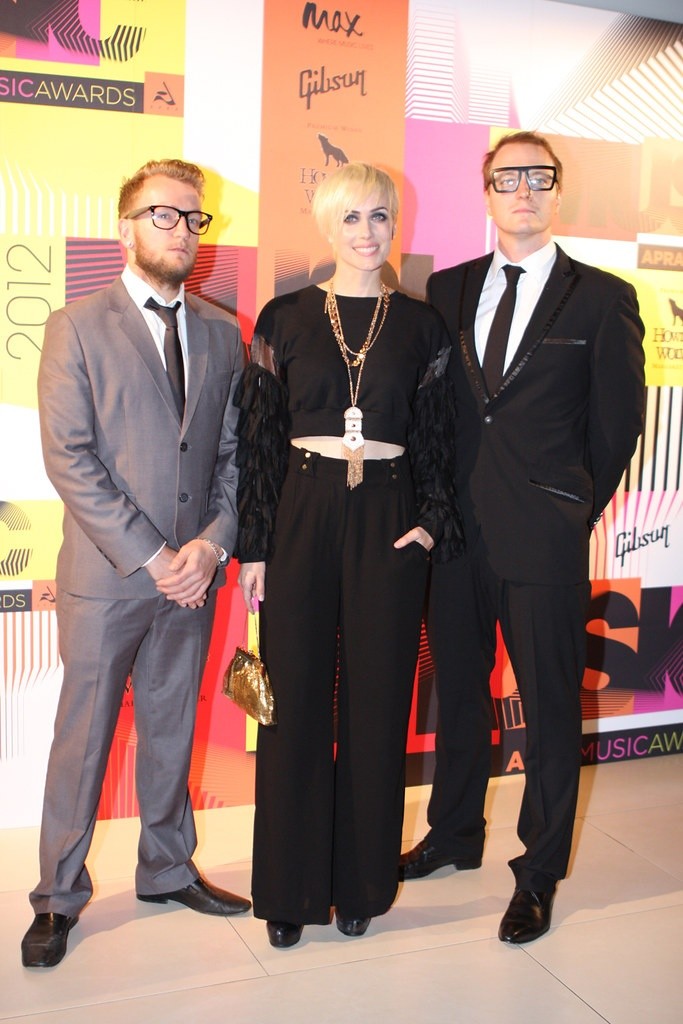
[197,537,221,567]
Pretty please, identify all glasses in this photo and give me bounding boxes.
[485,165,559,193]
[132,205,212,235]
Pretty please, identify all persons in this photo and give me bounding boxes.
[21,160,255,970]
[231,166,424,946]
[394,130,646,940]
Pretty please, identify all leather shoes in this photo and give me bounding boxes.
[136,876,251,917]
[399,839,483,882]
[266,917,305,948]
[334,910,372,936]
[21,912,79,967]
[498,887,557,944]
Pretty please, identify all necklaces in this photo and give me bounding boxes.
[322,277,395,486]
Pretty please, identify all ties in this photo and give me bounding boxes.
[143,294,186,426]
[481,264,526,401]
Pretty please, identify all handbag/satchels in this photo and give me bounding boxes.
[221,596,279,728]
